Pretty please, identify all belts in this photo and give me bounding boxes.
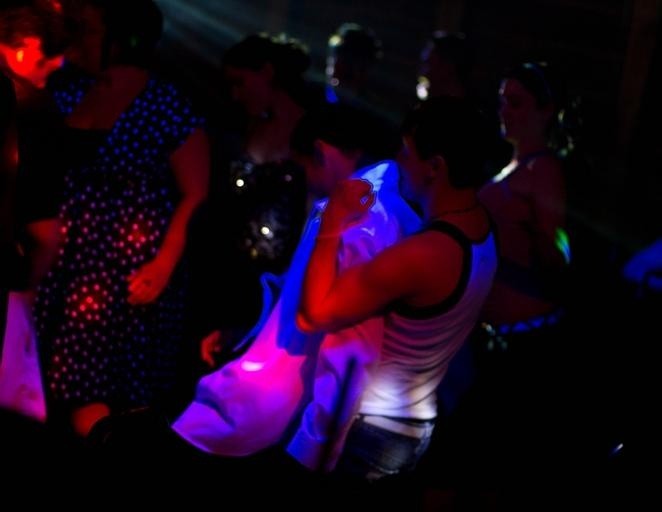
[352,412,436,443]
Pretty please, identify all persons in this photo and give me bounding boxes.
[1,1,580,487]
